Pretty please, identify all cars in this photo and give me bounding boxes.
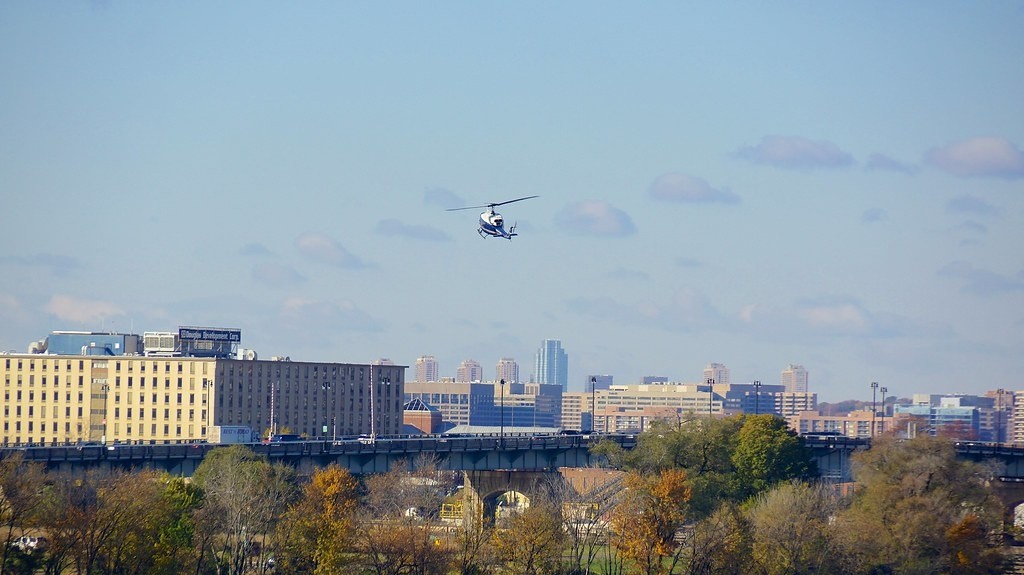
[559,428,607,436]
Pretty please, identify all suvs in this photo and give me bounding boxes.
[12,535,47,553]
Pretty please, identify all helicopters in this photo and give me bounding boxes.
[445,195,544,241]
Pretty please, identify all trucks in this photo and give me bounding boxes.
[208,425,262,445]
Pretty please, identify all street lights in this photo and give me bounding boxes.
[101,380,110,447]
[500,378,506,440]
[753,378,762,415]
[880,386,888,435]
[204,379,213,438]
[592,377,597,432]
[871,382,880,438]
[707,377,715,417]
